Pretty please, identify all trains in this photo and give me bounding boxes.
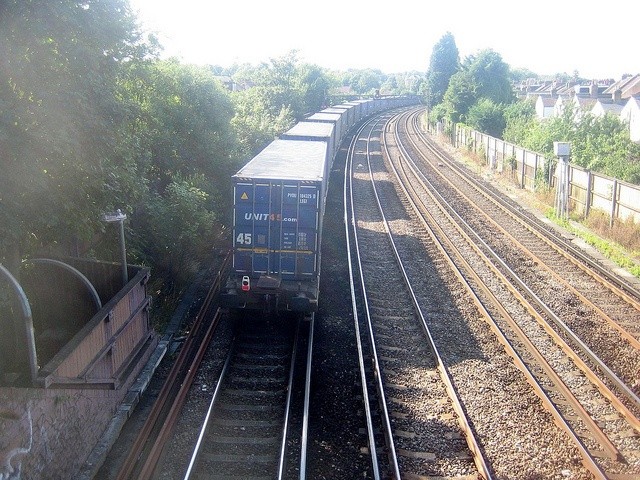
[218,95,419,333]
